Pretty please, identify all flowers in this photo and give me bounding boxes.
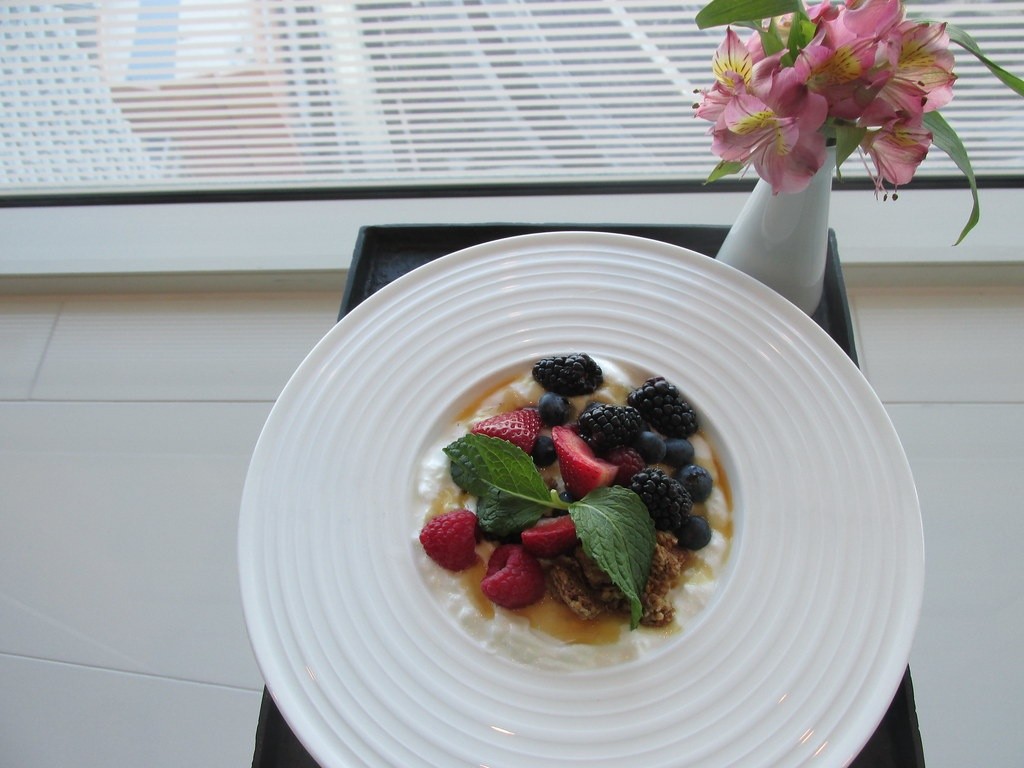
[692,0,1024,246]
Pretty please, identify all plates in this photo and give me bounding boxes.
[238,233,924,768]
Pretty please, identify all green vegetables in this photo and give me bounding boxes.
[441,434,656,632]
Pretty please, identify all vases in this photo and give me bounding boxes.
[713,142,836,319]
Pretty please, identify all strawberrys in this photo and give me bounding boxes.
[470,408,540,453]
[552,427,620,501]
